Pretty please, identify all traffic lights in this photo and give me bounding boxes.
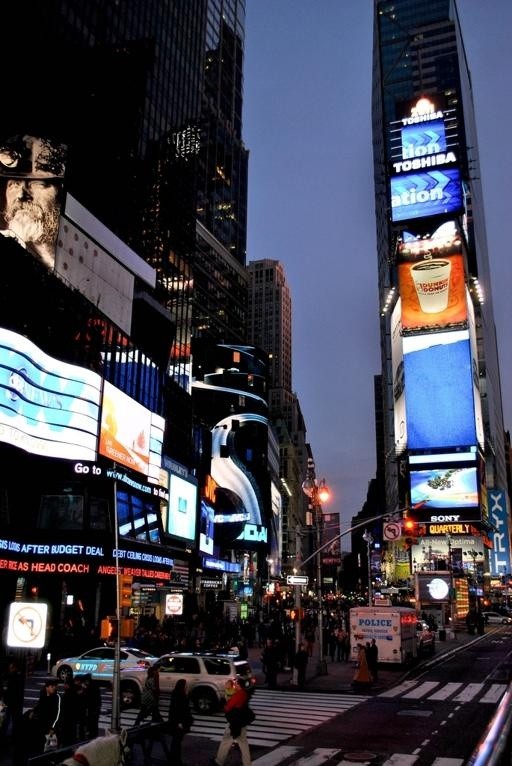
[414,523,420,544]
[405,521,413,544]
[290,610,297,621]
[301,609,304,620]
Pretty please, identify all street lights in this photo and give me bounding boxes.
[361,529,374,608]
[463,548,483,635]
[291,497,430,686]
[501,565,508,615]
[267,558,273,622]
[108,459,131,732]
[300,470,331,677]
[444,527,456,642]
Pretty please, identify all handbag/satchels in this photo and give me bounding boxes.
[44,733,59,754]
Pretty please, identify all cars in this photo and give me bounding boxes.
[49,646,161,684]
[480,612,512,625]
[416,620,436,647]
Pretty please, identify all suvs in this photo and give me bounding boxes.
[119,651,257,715]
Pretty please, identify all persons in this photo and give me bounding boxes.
[2,671,192,764]
[2,589,487,685]
[215,678,254,764]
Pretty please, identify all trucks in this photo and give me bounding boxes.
[348,598,418,666]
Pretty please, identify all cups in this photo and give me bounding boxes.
[410,258,451,315]
